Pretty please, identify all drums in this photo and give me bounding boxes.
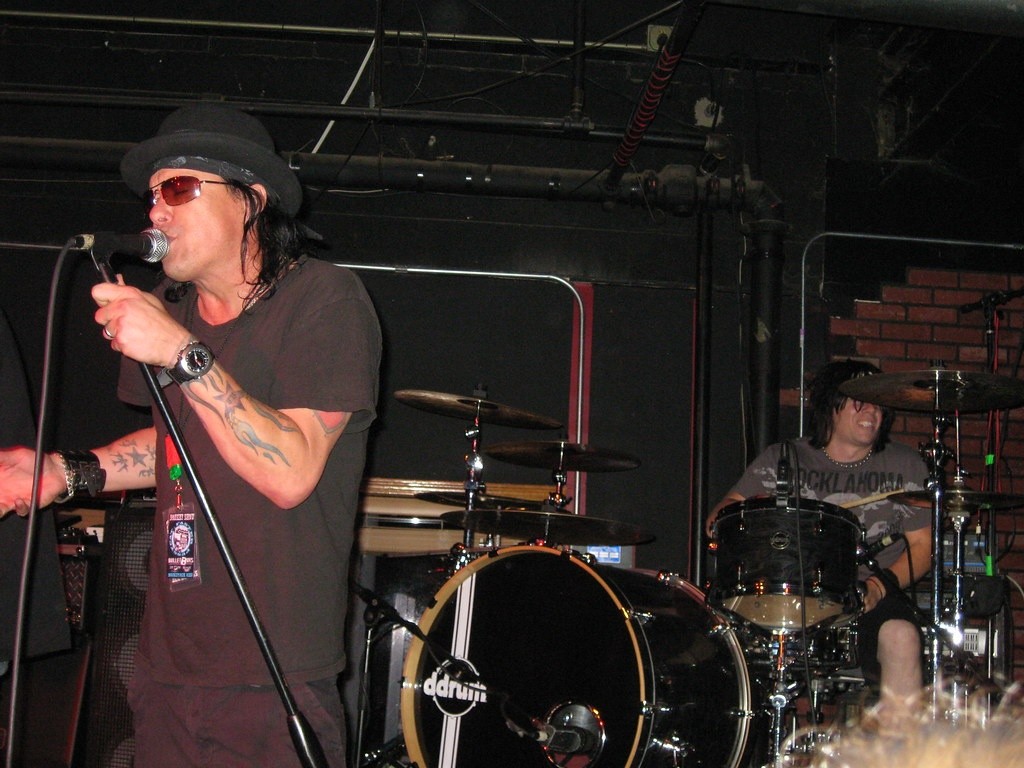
[707,493,868,632]
[398,544,753,768]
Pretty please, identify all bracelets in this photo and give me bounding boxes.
[869,568,901,598]
[53,449,107,505]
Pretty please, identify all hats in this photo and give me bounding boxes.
[120,103,304,218]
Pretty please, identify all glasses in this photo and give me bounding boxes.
[140,175,230,216]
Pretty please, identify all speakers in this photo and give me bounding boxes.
[81,504,162,768]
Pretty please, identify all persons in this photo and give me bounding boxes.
[0,104,382,768]
[703,359,934,706]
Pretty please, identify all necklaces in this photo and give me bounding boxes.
[821,446,876,467]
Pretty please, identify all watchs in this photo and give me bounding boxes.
[153,339,216,391]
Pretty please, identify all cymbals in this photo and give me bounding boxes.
[884,486,1023,511]
[435,504,656,545]
[392,387,565,429]
[477,436,642,474]
[837,366,1024,413]
[412,487,574,515]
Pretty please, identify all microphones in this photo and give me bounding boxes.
[856,532,903,565]
[68,228,169,263]
[519,726,597,753]
[777,440,792,506]
